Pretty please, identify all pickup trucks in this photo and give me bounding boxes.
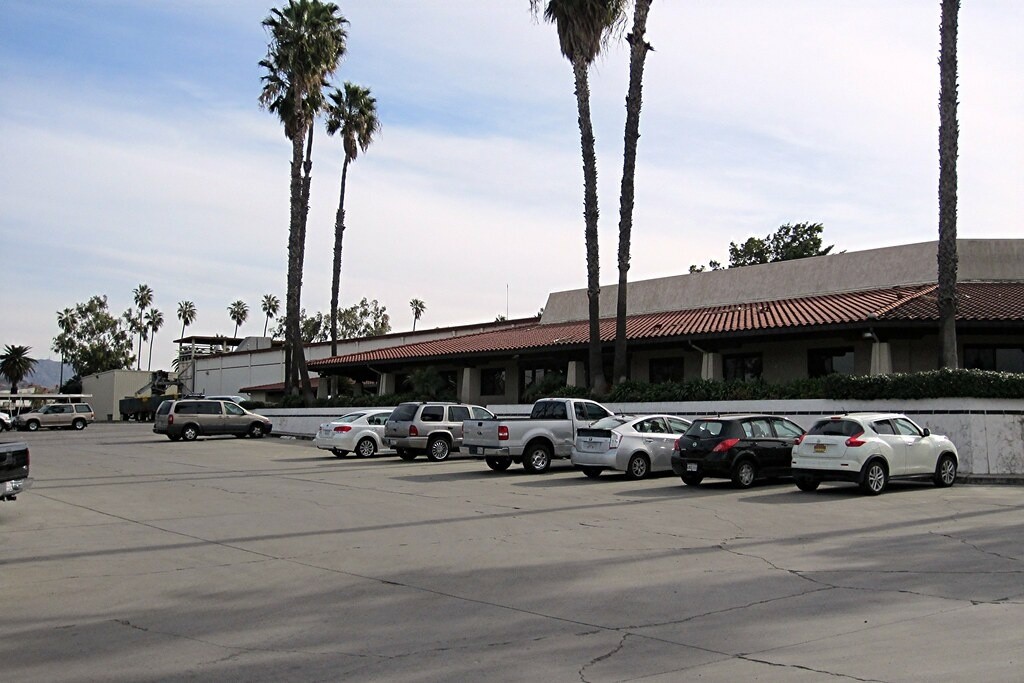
[0,440,33,500]
[458,398,617,476]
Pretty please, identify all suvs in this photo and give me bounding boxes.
[207,395,247,407]
[151,399,274,444]
[0,400,96,432]
[382,402,498,460]
[789,414,960,495]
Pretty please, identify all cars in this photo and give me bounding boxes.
[569,411,712,479]
[312,408,398,459]
[670,414,810,487]
